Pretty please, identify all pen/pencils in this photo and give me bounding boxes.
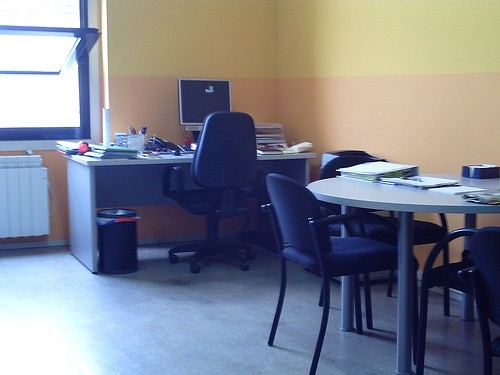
[400,176,421,181]
[128,125,147,135]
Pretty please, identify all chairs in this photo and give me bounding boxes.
[265,173,420,375]
[161,111,268,274]
[416,226,500,375]
[319,154,451,330]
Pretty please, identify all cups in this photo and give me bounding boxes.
[127,134,144,153]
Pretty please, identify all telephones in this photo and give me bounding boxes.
[147,137,177,151]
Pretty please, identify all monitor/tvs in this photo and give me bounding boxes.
[177,78,231,142]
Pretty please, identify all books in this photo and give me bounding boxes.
[57,120,291,158]
[337,162,487,196]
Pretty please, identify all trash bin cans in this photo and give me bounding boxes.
[96,207,142,275]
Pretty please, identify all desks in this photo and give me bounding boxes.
[306,173,500,375]
[59,152,319,274]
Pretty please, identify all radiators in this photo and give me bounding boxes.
[0,154,55,240]
